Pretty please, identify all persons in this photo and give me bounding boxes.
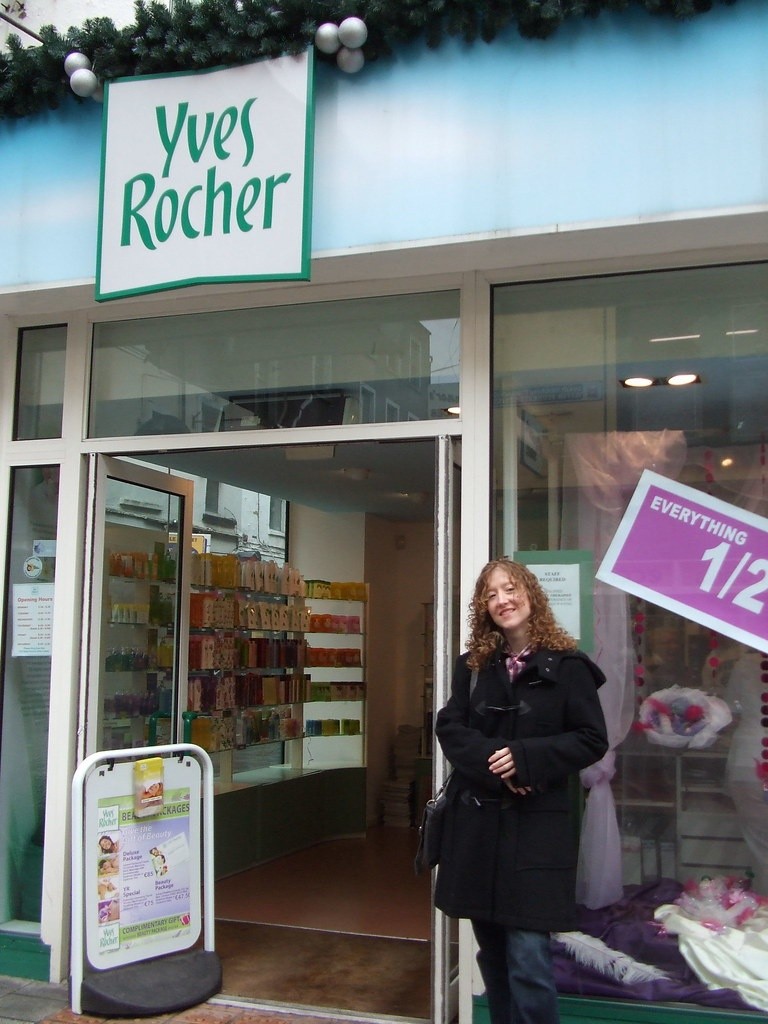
[99,835,118,853]
[433,558,609,1024]
[142,782,163,799]
[99,858,118,875]
[150,847,167,876]
[99,883,115,900]
[107,899,119,921]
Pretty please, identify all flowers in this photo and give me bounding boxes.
[676,870,763,932]
[633,694,706,735]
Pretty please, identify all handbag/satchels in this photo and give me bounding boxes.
[415,794,447,876]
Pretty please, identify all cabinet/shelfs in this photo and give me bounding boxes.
[615,600,768,893]
[415,601,433,834]
[101,554,370,888]
